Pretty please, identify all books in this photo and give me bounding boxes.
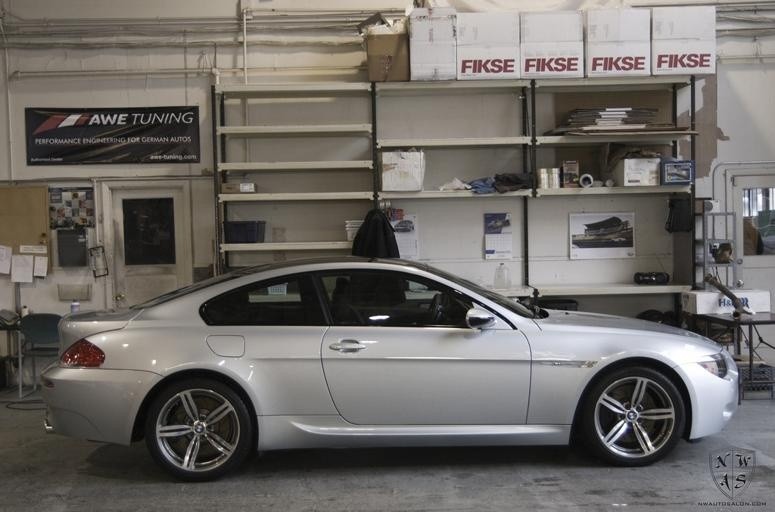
[564,106,659,126]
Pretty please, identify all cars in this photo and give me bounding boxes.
[394,220,414,232]
[39,255,740,484]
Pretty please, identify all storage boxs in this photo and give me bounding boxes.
[680,284,771,317]
[381,147,426,192]
[600,149,695,189]
[356,0,716,81]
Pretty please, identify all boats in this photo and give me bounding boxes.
[572,217,633,248]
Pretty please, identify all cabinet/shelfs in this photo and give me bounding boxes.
[209,76,699,328]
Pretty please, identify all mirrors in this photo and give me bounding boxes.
[733,173,775,261]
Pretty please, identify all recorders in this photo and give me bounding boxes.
[633,272,669,285]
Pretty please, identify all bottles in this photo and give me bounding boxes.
[21,305,28,319]
[494,263,510,288]
[71,298,80,314]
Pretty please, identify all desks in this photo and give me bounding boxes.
[706,312,774,403]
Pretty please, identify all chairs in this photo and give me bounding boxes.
[5,314,62,399]
